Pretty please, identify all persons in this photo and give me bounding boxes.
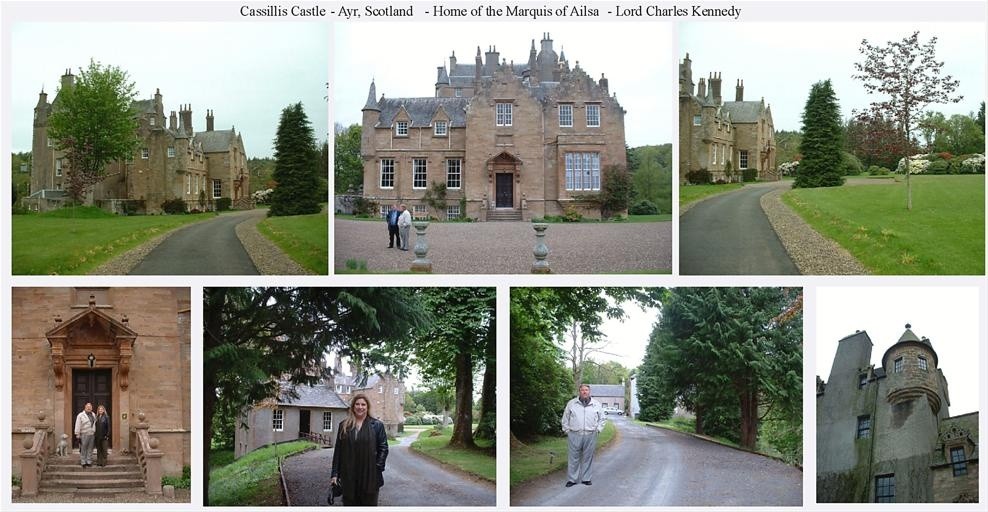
[331,394,389,506]
[397,204,411,250]
[94,405,111,466]
[561,384,606,487]
[386,204,402,249]
[75,403,97,465]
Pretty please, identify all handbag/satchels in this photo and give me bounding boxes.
[333,481,343,497]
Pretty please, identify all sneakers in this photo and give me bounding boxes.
[582,481,592,485]
[566,481,576,487]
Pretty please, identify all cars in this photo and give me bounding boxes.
[603,407,624,415]
